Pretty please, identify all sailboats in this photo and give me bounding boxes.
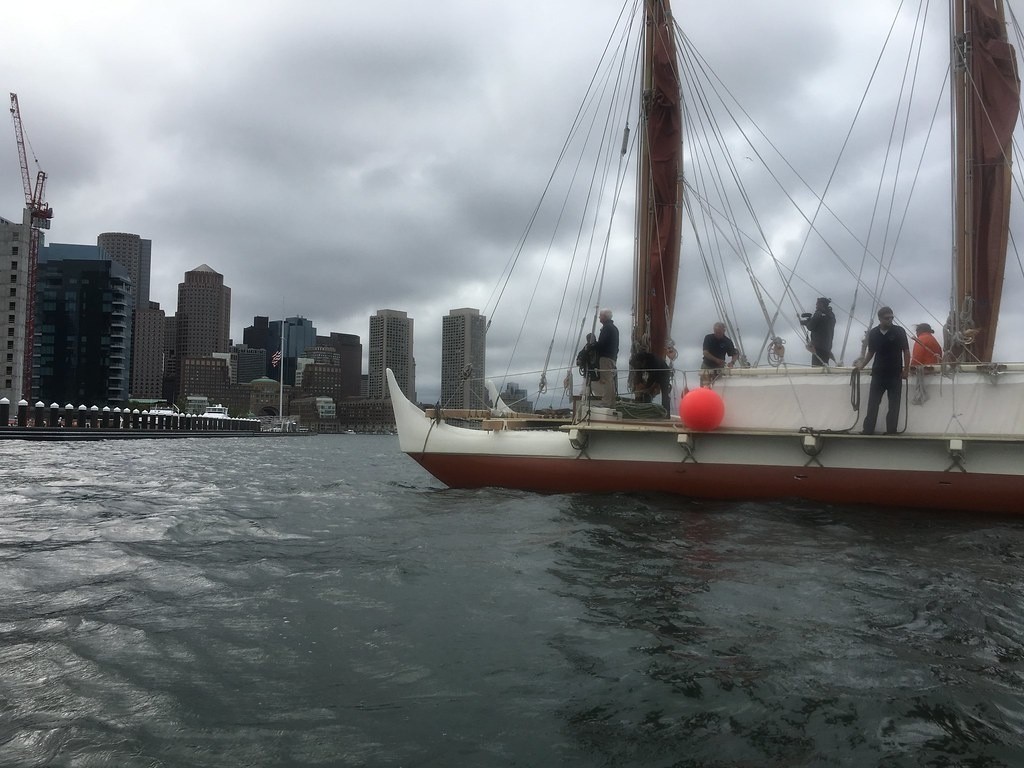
[385,0,1023,520]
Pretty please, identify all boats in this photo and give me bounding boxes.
[202,404,230,419]
[148,399,180,416]
[344,429,357,435]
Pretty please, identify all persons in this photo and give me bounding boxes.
[804,298,836,367]
[700,322,739,387]
[911,323,942,365]
[576,333,601,396]
[598,310,619,401]
[854,307,910,435]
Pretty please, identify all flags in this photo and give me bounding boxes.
[272,351,282,368]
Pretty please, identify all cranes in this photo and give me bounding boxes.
[10,92,55,426]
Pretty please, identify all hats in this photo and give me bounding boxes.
[914,323,935,334]
[878,307,894,318]
